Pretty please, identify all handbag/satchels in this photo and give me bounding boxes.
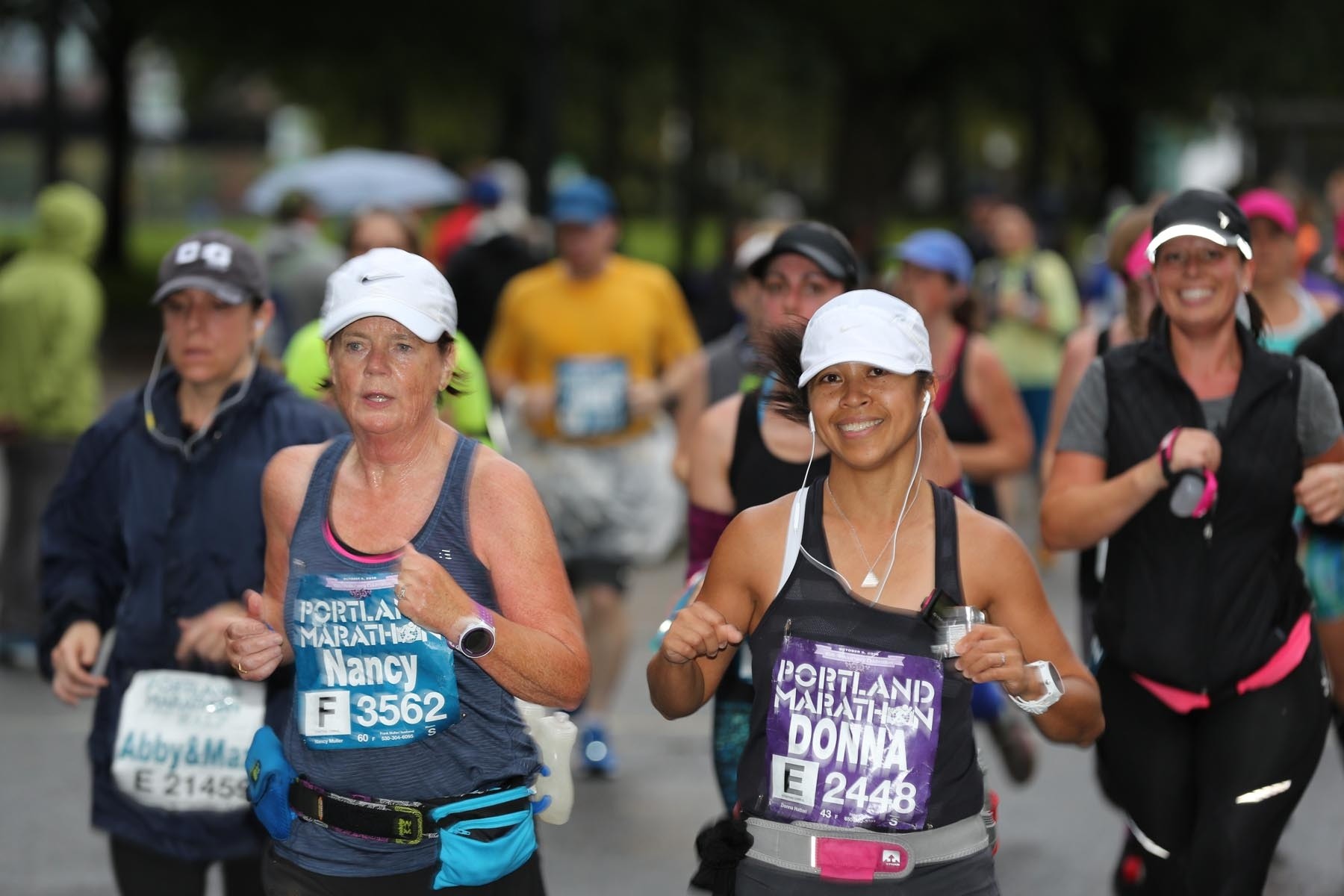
[432,785,538,892]
[244,725,297,839]
[110,668,266,815]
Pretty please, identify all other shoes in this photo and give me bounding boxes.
[579,724,620,781]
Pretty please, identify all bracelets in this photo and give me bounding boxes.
[1158,425,1187,480]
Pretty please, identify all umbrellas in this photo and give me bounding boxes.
[236,150,472,217]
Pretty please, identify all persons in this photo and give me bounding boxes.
[0,149,1344,896]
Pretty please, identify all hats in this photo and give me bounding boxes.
[746,220,858,286]
[889,227,973,283]
[153,232,261,308]
[1143,187,1253,264]
[797,288,933,389]
[319,247,459,344]
[551,181,610,225]
[1234,189,1299,237]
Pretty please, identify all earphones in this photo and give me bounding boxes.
[808,412,817,432]
[921,392,933,417]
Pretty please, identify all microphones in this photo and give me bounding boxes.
[255,313,265,337]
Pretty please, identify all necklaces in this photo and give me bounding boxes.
[826,473,923,586]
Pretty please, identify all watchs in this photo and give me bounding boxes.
[1006,660,1065,715]
[448,603,496,660]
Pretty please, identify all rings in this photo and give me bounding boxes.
[238,663,249,674]
[999,652,1005,666]
[398,588,406,599]
